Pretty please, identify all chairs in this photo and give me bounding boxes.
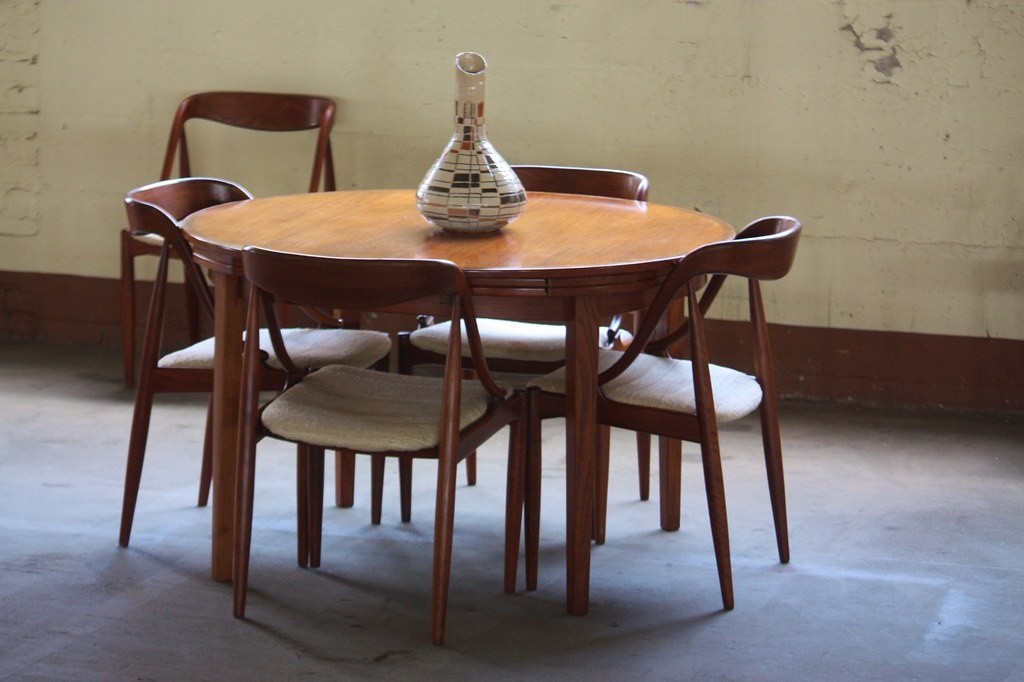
[522,216,804,615]
[398,161,651,536]
[121,91,340,393]
[233,246,530,641]
[118,176,390,566]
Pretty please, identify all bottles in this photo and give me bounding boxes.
[413,50,523,236]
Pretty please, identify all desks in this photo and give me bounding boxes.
[182,187,735,616]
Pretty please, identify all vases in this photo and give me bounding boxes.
[418,55,528,237]
